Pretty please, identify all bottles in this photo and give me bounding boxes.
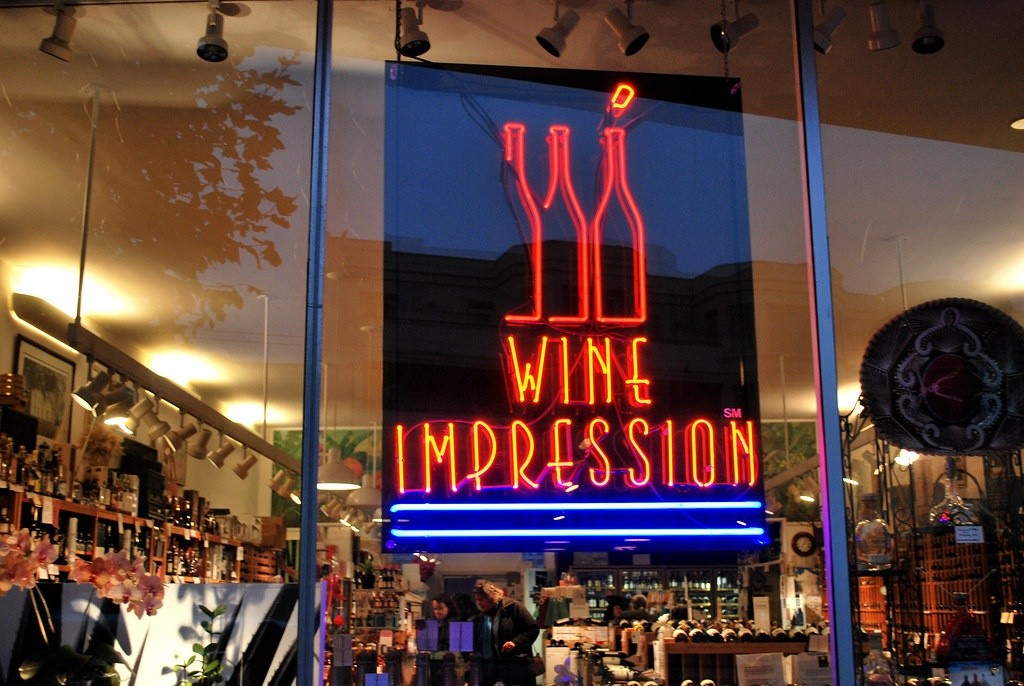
[930,474,979,525]
[626,679,716,686]
[584,575,608,586]
[322,639,378,686]
[622,571,741,589]
[572,642,651,681]
[585,616,607,625]
[616,617,652,632]
[586,588,610,608]
[854,492,894,571]
[671,621,830,641]
[0,433,219,535]
[554,615,581,625]
[863,634,892,685]
[551,639,567,647]
[350,564,401,589]
[348,594,408,629]
[935,593,984,665]
[1,503,238,582]
[675,592,738,619]
[591,612,605,619]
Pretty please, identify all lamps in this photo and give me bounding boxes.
[912,0,945,55]
[603,0,650,56]
[40,0,77,62]
[207,431,235,468]
[866,0,900,52]
[843,457,865,485]
[232,444,258,479]
[813,0,848,55]
[317,271,362,492]
[535,0,581,58]
[165,409,197,452]
[197,0,229,62]
[152,428,188,486]
[800,490,816,502]
[710,0,759,53]
[186,422,212,459]
[318,493,377,535]
[72,355,170,441]
[267,462,301,505]
[399,0,430,57]
[345,325,382,507]
[860,449,889,475]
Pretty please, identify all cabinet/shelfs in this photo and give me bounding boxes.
[820,522,1024,666]
[350,588,408,632]
[0,480,242,583]
[568,564,740,621]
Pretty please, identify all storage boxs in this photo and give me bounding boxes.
[209,508,287,549]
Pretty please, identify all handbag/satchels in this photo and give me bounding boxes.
[528,655,546,676]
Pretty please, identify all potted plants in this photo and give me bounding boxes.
[358,558,379,589]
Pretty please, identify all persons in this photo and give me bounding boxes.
[614,595,652,626]
[472,579,540,686]
[428,593,461,686]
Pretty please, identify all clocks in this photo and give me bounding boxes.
[792,531,817,556]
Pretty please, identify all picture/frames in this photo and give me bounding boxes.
[14,331,77,499]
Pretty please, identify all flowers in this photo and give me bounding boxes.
[0,523,168,686]
